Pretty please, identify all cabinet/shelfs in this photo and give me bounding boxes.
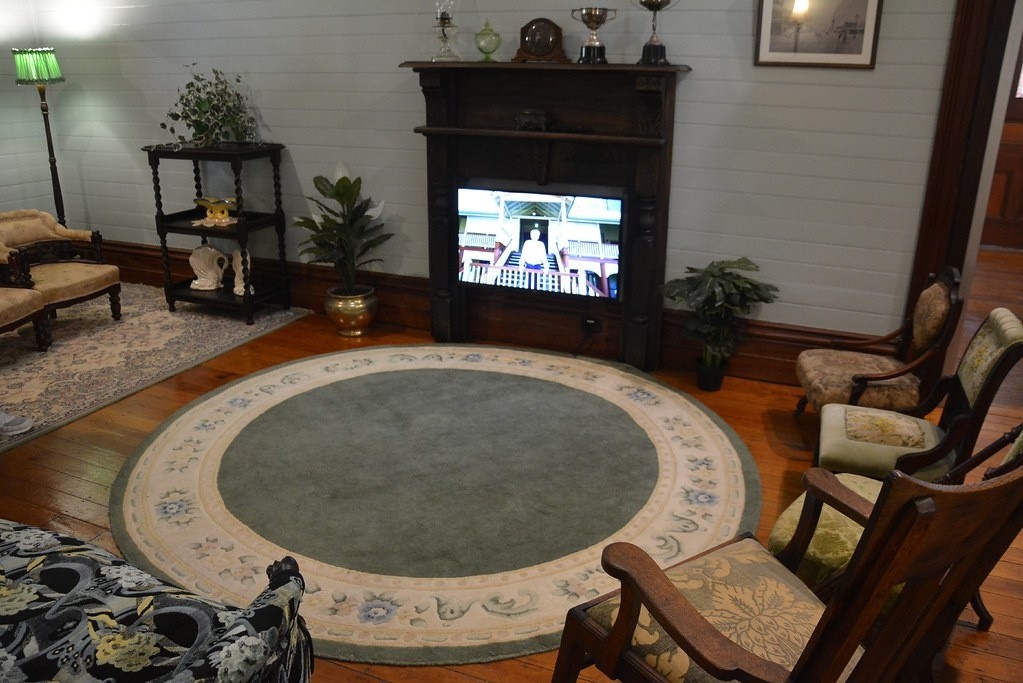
[399,61,690,365]
[141,137,291,324]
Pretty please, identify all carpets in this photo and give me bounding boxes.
[0,282,316,452]
[103,340,764,669]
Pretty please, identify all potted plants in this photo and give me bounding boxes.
[150,60,272,151]
[667,257,780,392]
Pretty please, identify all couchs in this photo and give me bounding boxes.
[0,517,313,683]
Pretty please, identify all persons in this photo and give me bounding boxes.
[519,229,549,290]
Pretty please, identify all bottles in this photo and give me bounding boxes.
[473,17,501,62]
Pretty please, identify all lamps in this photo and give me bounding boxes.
[12,45,68,227]
[293,175,397,335]
[432,0,465,62]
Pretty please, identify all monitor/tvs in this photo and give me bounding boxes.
[451,175,628,305]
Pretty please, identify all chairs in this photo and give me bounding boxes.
[550,269,1023,683]
[0,209,124,351]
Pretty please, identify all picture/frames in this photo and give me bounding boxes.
[755,0,883,70]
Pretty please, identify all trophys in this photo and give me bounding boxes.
[571,7,617,63]
[630,0,681,65]
